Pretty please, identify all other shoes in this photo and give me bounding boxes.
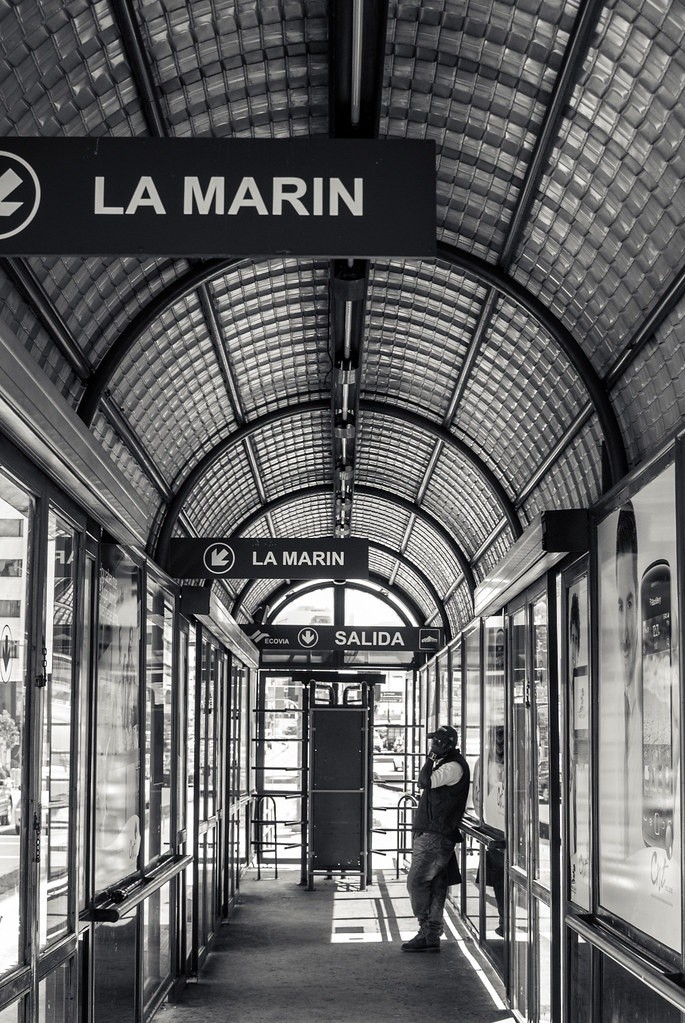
[400,931,441,952]
[495,924,504,938]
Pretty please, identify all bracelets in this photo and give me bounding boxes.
[428,750,437,761]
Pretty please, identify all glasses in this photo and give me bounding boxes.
[431,737,447,747]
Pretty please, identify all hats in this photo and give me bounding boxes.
[427,725,458,745]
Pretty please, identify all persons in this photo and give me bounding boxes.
[566,592,580,856]
[402,725,471,954]
[472,725,508,938]
[611,501,643,861]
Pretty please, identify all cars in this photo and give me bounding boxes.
[374,732,382,753]
[393,746,421,772]
[0,764,54,834]
[538,761,562,801]
[384,736,405,752]
